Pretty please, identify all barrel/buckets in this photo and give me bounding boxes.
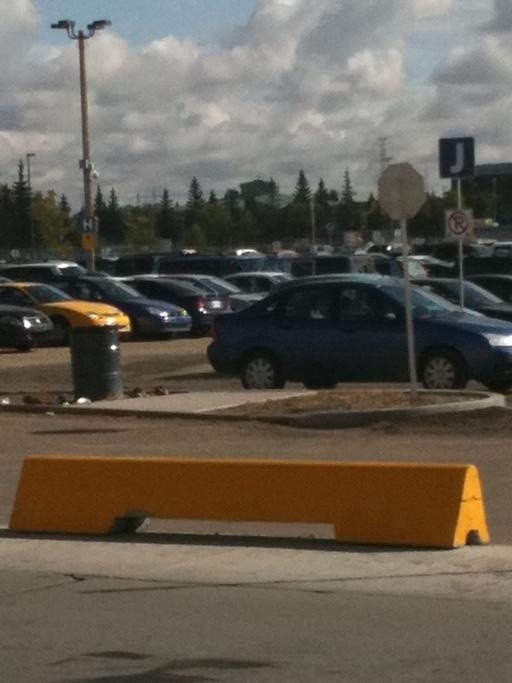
[69,326,124,401]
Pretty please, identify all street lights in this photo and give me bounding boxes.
[46,16,115,276]
[23,152,36,256]
